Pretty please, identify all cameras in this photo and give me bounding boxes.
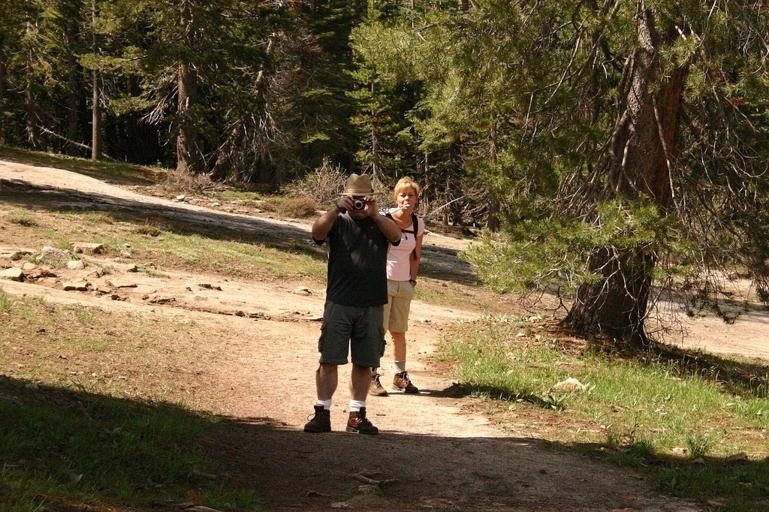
[348,199,365,210]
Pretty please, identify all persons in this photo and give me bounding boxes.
[304,171,402,436]
[368,177,425,398]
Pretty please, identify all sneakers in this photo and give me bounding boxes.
[346,408,378,434]
[368,373,387,396]
[391,371,418,394]
[304,405,331,433]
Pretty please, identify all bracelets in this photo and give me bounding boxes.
[409,279,417,284]
[333,201,346,214]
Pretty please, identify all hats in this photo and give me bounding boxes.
[338,172,373,196]
